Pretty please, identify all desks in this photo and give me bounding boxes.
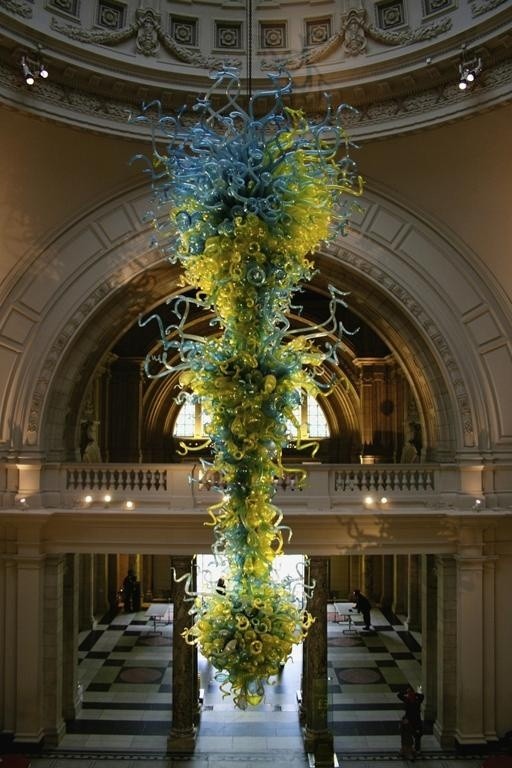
[332,603,362,636]
[143,602,171,637]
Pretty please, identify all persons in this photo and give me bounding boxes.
[123,569,135,613]
[400,717,420,754]
[352,589,372,630]
[398,687,424,754]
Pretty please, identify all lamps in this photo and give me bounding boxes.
[21,49,52,86]
[456,53,483,91]
[120,0,368,714]
